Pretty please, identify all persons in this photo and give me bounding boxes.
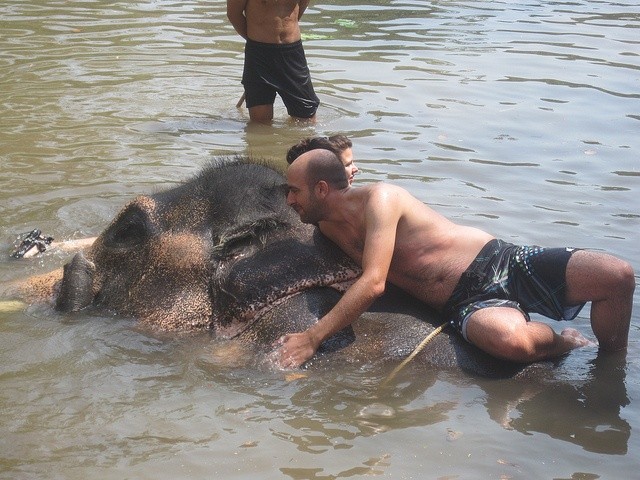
[227,0,320,123]
[12,134,359,260]
[266,136,635,371]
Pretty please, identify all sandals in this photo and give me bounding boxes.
[13,227,54,261]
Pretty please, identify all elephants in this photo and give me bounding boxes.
[0,153,629,393]
